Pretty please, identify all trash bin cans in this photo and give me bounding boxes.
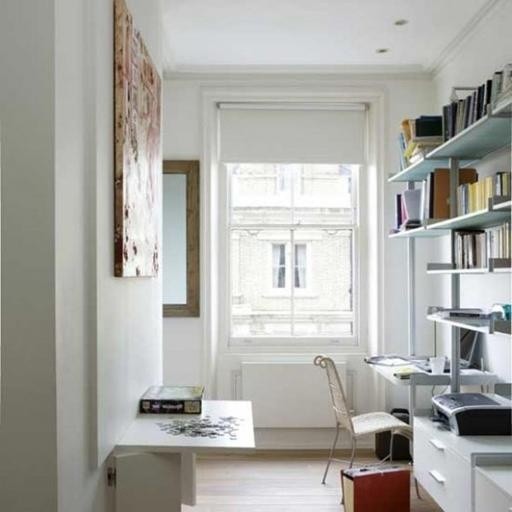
[375,408,409,461]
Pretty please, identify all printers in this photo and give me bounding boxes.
[431,392,512,435]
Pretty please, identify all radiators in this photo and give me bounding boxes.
[242,361,346,429]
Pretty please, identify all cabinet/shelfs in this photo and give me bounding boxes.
[389,94,512,512]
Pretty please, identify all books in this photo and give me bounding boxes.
[455,172,511,217]
[395,168,478,231]
[442,64,512,142]
[139,385,205,414]
[451,222,511,269]
[437,308,485,319]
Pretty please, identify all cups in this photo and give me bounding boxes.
[430,357,445,374]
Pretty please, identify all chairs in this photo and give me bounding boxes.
[313,354,413,507]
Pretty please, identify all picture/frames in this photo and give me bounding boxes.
[114,0,161,279]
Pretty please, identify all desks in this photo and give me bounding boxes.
[110,400,256,512]
[365,354,499,458]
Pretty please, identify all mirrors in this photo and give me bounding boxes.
[161,159,200,321]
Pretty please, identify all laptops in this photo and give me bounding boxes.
[416,329,478,372]
